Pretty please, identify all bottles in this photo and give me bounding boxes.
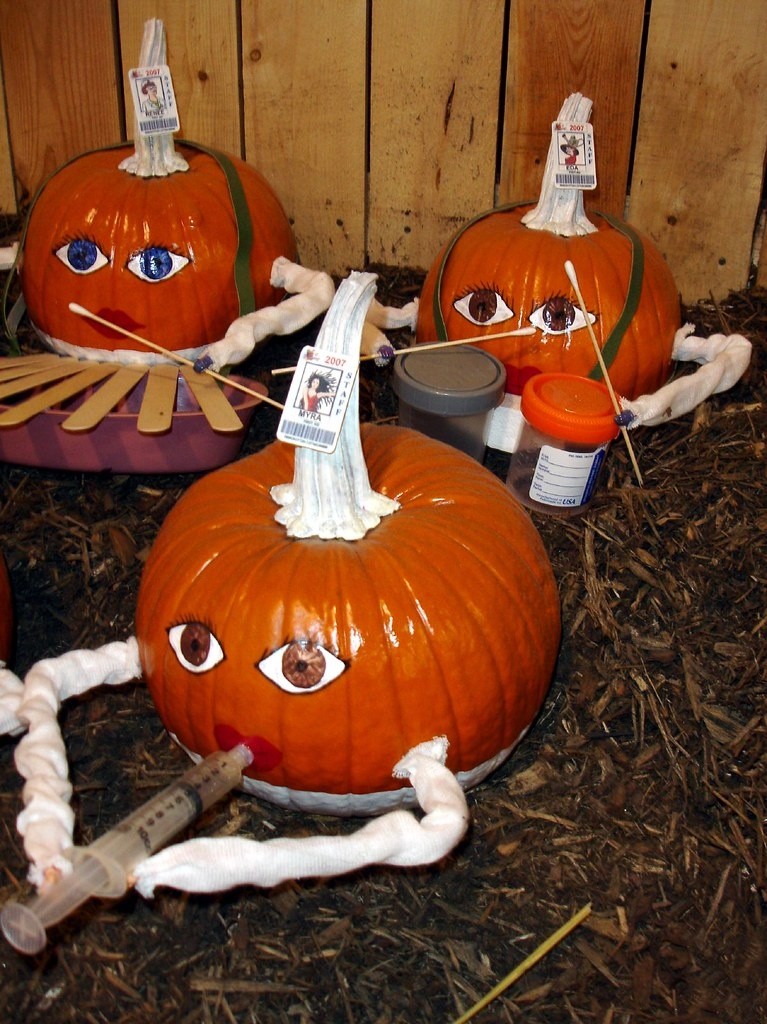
[509,374,623,514]
[389,344,506,459]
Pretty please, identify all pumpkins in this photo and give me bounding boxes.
[139,276,560,813]
[417,92,679,401]
[24,17,294,363]
